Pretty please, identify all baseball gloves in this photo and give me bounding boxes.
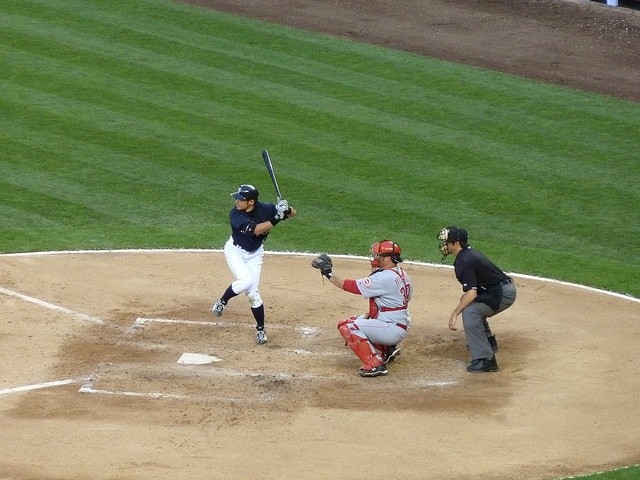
[311,253,334,279]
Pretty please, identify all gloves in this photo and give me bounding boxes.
[275,197,288,219]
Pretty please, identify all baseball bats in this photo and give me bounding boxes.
[262,150,289,214]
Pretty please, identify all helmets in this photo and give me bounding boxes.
[437,226,469,256]
[230,184,259,200]
[369,240,403,268]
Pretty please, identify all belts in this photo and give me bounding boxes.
[503,279,514,284]
[396,323,407,329]
[233,240,248,252]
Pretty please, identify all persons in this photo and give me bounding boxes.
[311,240,413,376]
[437,227,517,372]
[211,183,296,344]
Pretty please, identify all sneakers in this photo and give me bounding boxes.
[359,361,388,377]
[380,346,401,363]
[466,335,498,353]
[256,325,267,344]
[211,298,228,317]
[467,355,498,371]
